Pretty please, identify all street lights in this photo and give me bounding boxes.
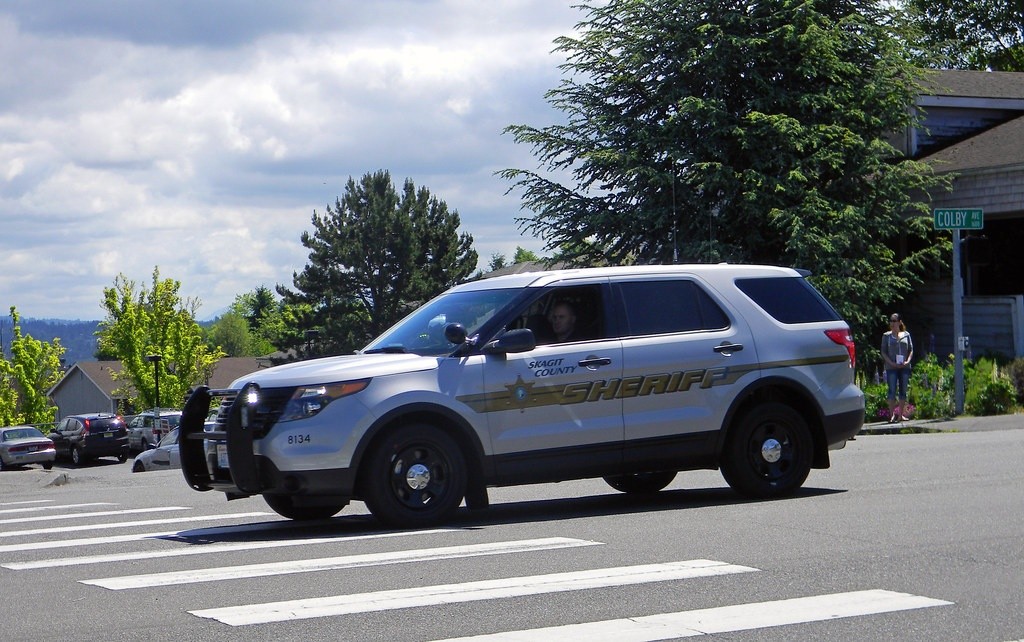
[148,354,162,447]
[304,330,319,358]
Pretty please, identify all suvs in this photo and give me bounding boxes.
[126,407,182,457]
[176,264,867,529]
[46,412,131,467]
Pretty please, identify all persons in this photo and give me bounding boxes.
[532,302,586,350]
[881,313,913,423]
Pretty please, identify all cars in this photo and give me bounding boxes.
[0,425,56,470]
[131,422,215,472]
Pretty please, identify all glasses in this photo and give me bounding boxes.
[889,320,899,322]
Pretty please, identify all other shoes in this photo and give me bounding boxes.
[898,415,909,421]
[888,415,896,423]
